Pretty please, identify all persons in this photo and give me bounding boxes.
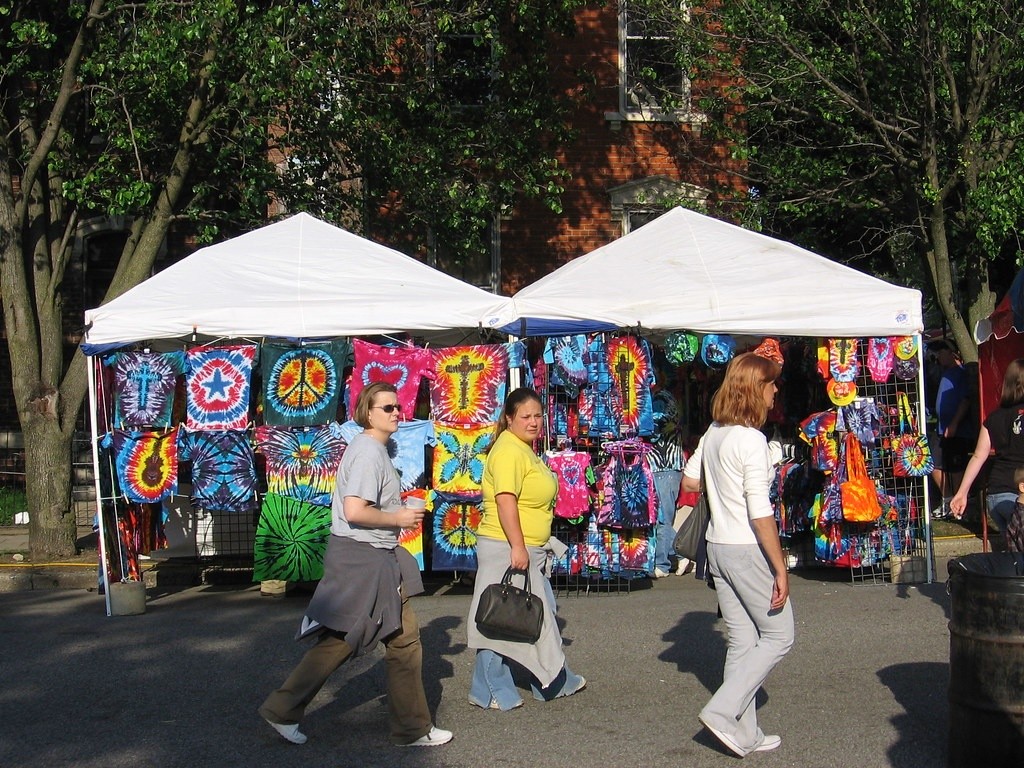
[258,382,453,748]
[925,344,969,524]
[677,350,797,756]
[467,387,587,711]
[950,358,1024,552]
[646,522,690,579]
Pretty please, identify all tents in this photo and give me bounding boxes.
[78,211,525,619]
[499,205,933,584]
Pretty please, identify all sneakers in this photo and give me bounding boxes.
[676,559,690,576]
[933,500,956,518]
[265,715,307,744]
[646,567,670,578]
[394,724,453,746]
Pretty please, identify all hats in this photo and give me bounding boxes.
[664,330,699,366]
[935,339,959,353]
[701,333,736,372]
[827,377,861,406]
[893,356,919,381]
[894,335,919,361]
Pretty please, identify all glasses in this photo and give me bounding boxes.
[368,405,402,413]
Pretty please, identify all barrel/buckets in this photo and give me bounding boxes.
[946,551,1024,768]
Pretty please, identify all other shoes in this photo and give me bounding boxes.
[533,674,586,701]
[469,698,525,710]
[751,735,781,752]
[698,718,746,758]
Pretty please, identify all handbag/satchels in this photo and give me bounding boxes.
[475,565,543,643]
[672,455,710,560]
[889,553,926,585]
[817,432,881,524]
[890,392,934,477]
